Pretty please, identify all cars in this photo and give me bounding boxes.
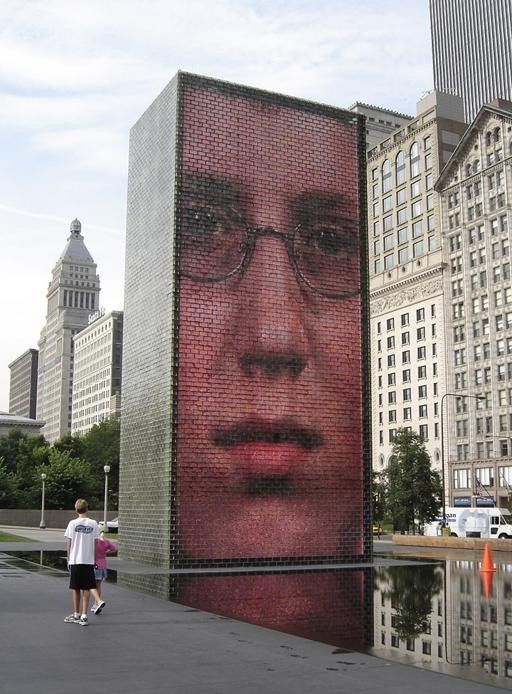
[99,517,118,530]
[372,524,382,535]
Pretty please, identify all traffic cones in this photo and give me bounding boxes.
[479,570,497,599]
[480,544,496,571]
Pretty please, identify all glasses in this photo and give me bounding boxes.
[177,188,362,301]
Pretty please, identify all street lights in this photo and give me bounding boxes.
[40,473,47,528]
[104,464,111,532]
[440,393,488,536]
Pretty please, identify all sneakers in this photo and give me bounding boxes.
[64,612,89,626]
[90,599,106,615]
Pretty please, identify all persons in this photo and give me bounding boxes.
[90,538,118,614]
[173,83,365,560]
[62,497,100,626]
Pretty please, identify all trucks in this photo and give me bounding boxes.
[425,507,512,539]
